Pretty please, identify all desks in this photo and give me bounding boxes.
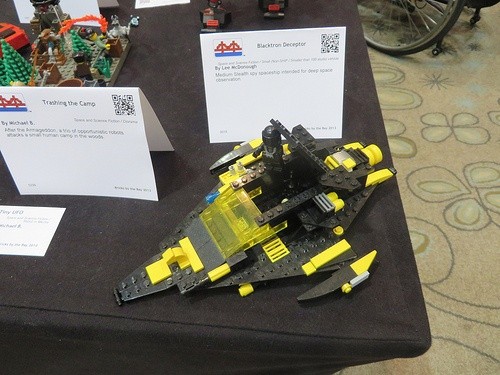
[0,0,435,375]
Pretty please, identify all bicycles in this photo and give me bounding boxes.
[355,1,499,56]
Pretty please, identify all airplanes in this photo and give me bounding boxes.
[112,115,397,310]
